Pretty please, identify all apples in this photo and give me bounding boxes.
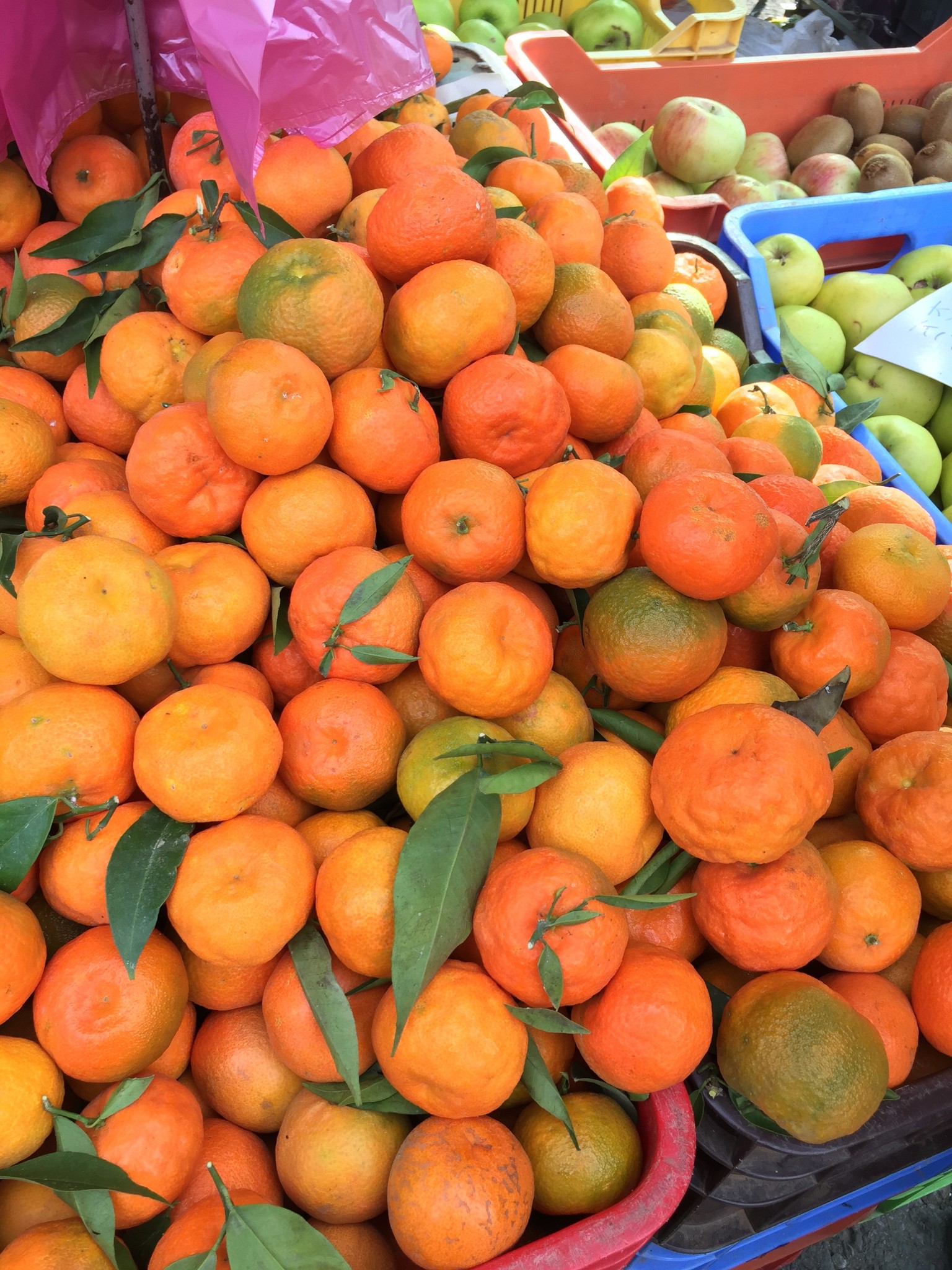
[409,1,646,55]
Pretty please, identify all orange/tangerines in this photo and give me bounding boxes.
[1,27,952,1270]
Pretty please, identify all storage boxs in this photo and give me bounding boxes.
[451,0,952,552]
[446,1029,950,1270]
